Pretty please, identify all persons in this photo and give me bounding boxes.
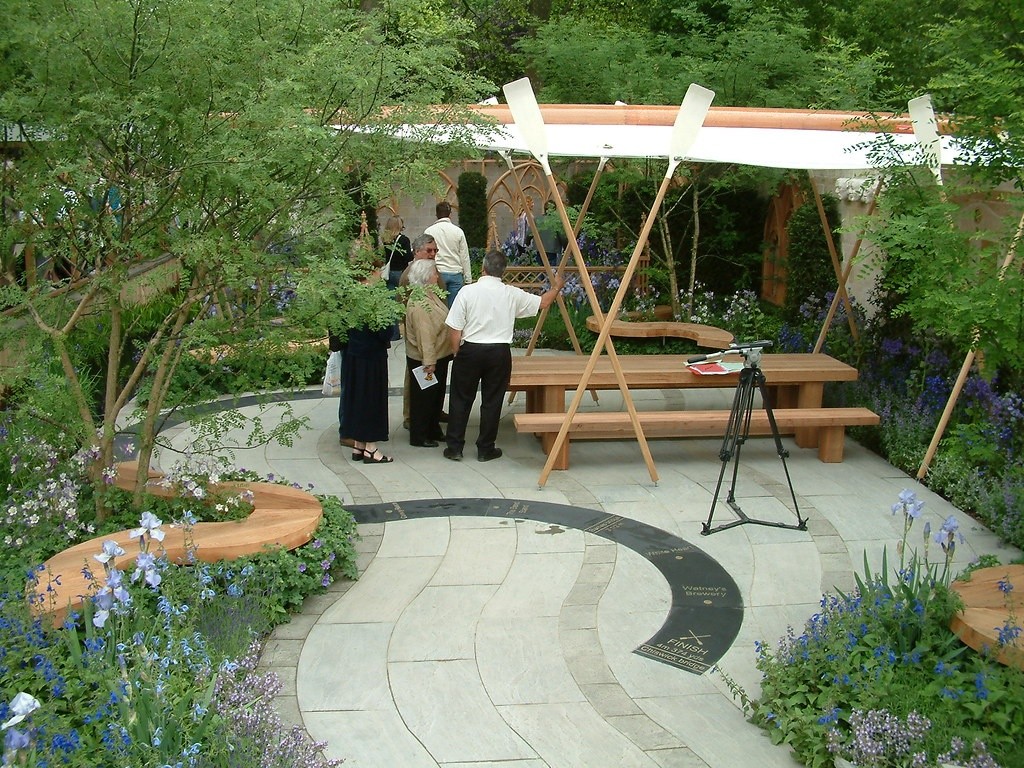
[330,255,394,463]
[515,195,568,266]
[0,153,195,310]
[384,202,473,447]
[444,250,564,462]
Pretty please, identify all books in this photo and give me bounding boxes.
[412,365,438,390]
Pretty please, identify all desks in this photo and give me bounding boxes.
[505,353,859,457]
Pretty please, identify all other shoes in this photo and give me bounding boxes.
[477,446,502,462]
[442,410,449,422]
[443,447,464,461]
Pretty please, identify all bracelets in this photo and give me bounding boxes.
[552,287,559,292]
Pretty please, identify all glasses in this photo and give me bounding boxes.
[426,247,439,253]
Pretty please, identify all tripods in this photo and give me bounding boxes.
[687,340,809,536]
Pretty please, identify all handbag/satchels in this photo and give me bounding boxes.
[322,352,342,396]
[379,262,390,280]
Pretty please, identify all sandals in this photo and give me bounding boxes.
[363,448,395,463]
[352,446,364,462]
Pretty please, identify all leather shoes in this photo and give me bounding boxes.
[436,435,446,440]
[411,437,439,447]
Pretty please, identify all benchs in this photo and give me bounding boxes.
[511,407,881,470]
